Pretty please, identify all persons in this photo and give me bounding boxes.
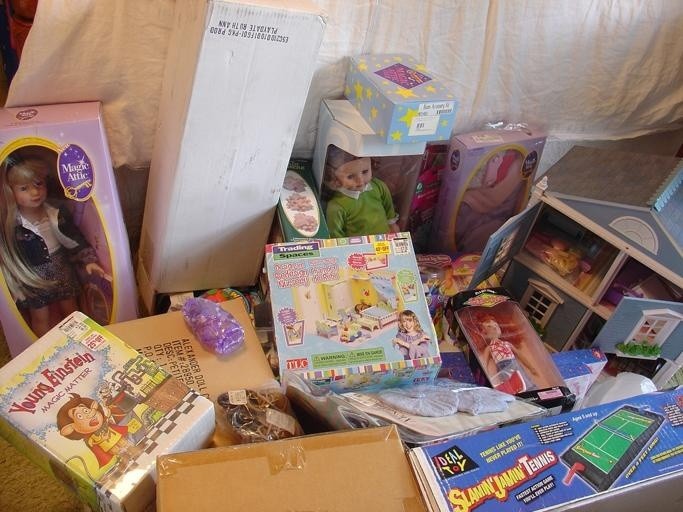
[327,151,402,240]
[392,309,431,360]
[468,312,539,392]
[0,156,108,338]
